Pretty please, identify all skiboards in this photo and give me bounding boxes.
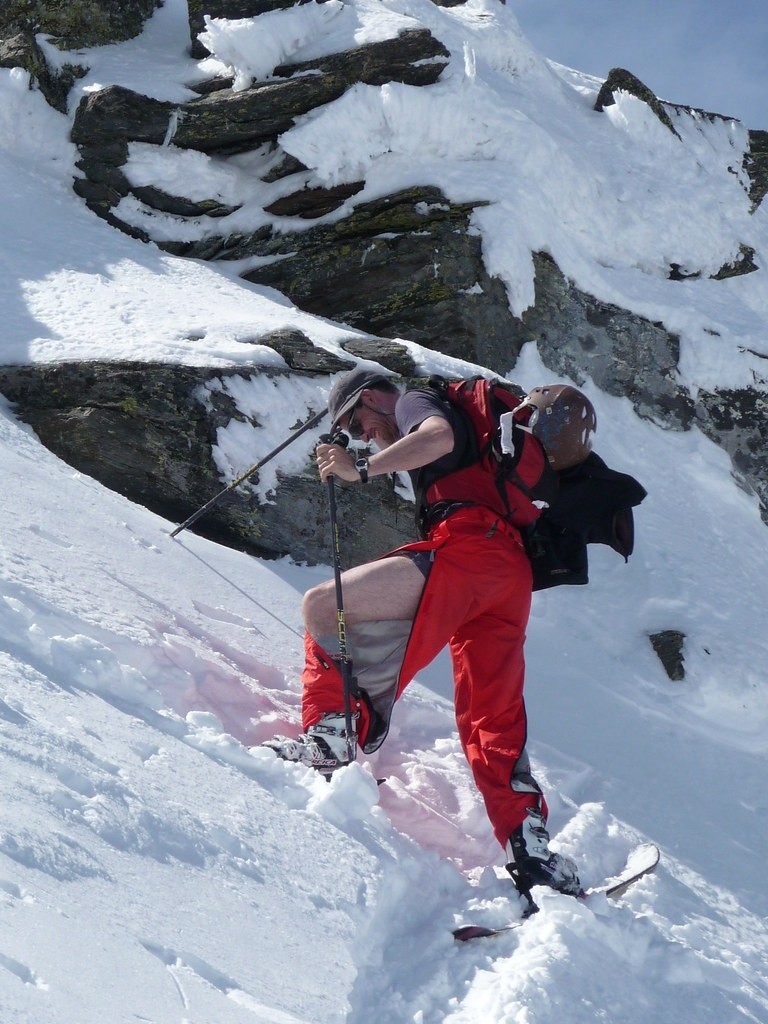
[453,842,664,940]
[303,766,390,793]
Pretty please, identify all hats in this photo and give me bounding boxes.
[327,368,386,440]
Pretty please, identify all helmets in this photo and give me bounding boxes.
[527,383,597,472]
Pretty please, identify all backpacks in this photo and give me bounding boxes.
[405,368,548,522]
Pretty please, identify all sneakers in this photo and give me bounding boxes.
[257,715,358,769]
[502,811,582,896]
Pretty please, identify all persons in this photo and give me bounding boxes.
[255,369,585,913]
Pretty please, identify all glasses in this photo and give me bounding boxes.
[347,409,364,440]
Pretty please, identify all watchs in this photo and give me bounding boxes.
[354,457,368,482]
